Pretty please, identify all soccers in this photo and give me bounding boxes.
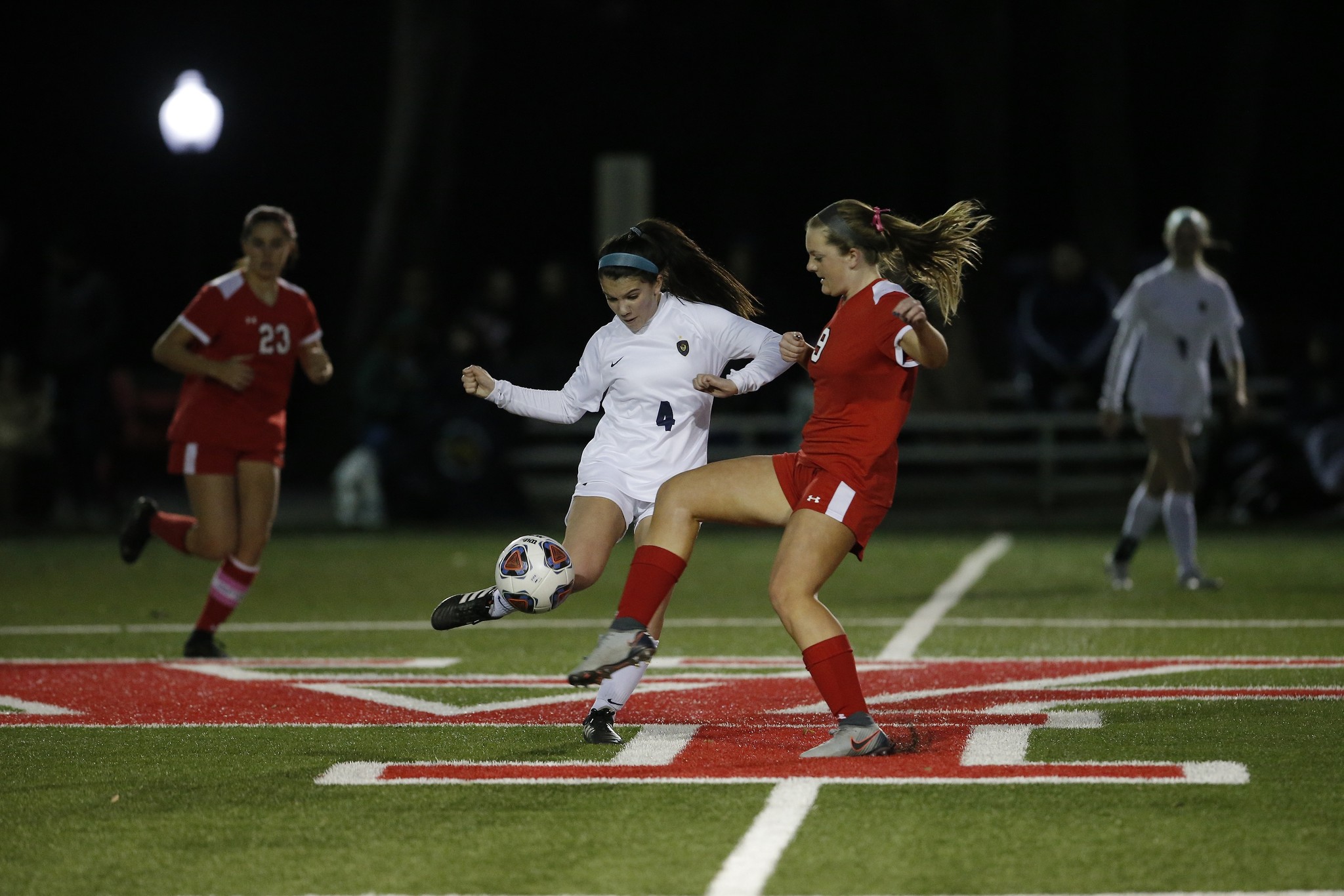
[494,533,576,613]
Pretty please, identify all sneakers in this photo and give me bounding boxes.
[431,586,504,631]
[1179,572,1218,592]
[1104,554,1133,588]
[799,723,893,760]
[120,496,158,567]
[582,707,625,745]
[569,629,657,686]
[184,634,228,658]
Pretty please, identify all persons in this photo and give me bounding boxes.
[0,236,774,482]
[116,202,337,662]
[563,195,991,760]
[1018,223,1121,413]
[1095,203,1248,591]
[426,214,796,749]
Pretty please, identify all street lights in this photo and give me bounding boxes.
[153,69,226,329]
